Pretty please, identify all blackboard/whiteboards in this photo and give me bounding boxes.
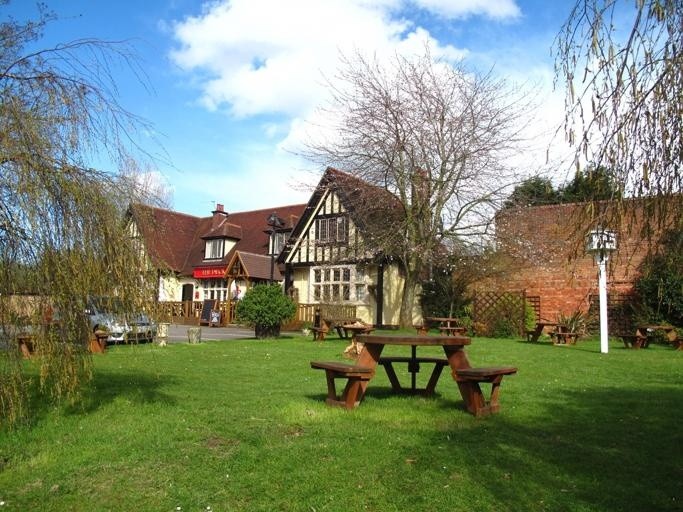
[199,299,220,326]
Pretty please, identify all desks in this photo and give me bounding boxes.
[340,333,487,416]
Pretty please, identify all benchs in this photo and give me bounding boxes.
[307,317,377,344]
[374,354,449,394]
[618,325,682,350]
[412,315,468,336]
[521,321,578,346]
[309,356,372,412]
[455,362,519,417]
[12,327,108,359]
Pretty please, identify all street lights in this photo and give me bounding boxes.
[262,210,285,285]
[581,220,622,354]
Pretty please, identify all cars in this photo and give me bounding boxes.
[54,295,157,348]
